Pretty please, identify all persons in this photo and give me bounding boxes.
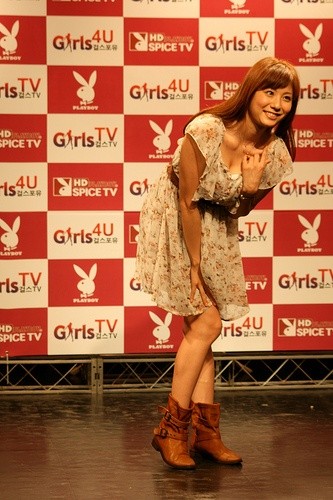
[133,56,301,469]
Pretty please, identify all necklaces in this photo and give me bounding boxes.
[236,120,269,156]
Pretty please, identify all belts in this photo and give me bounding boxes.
[165,167,232,212]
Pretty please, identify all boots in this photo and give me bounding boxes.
[152,392,197,470]
[191,401,244,466]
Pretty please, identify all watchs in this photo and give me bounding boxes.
[240,192,254,201]
